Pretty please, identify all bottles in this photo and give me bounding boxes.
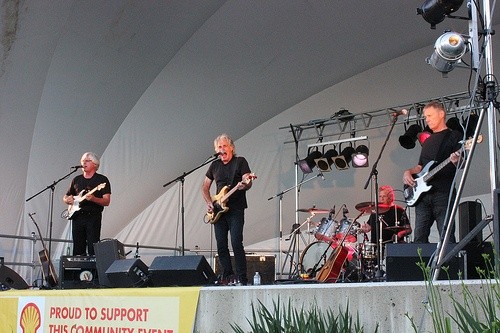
[254,272,261,285]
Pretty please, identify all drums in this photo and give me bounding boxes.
[360,242,378,262]
[335,218,361,243]
[299,240,359,275]
[313,216,340,243]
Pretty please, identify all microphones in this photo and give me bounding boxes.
[392,109,407,116]
[71,165,83,168]
[320,170,324,180]
[209,150,225,157]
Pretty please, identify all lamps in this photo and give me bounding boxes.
[446,101,483,144]
[424,30,473,78]
[416,0,472,29]
[296,136,371,174]
[398,109,433,149]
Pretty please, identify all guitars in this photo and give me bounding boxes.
[402,134,484,207]
[315,209,366,285]
[27,212,59,288]
[206,173,258,225]
[65,182,107,220]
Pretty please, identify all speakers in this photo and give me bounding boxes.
[0,263,29,290]
[59,255,99,290]
[147,256,220,287]
[385,242,495,282]
[459,201,483,243]
[105,259,150,289]
[95,240,125,288]
[214,255,275,285]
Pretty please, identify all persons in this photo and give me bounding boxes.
[403,103,467,242]
[361,185,412,266]
[63,152,112,255]
[201,134,252,286]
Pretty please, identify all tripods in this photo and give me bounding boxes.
[268,174,376,283]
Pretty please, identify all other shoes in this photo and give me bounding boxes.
[228,280,247,286]
[219,279,230,284]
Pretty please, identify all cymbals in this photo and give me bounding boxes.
[354,201,390,214]
[383,225,412,235]
[297,208,330,214]
